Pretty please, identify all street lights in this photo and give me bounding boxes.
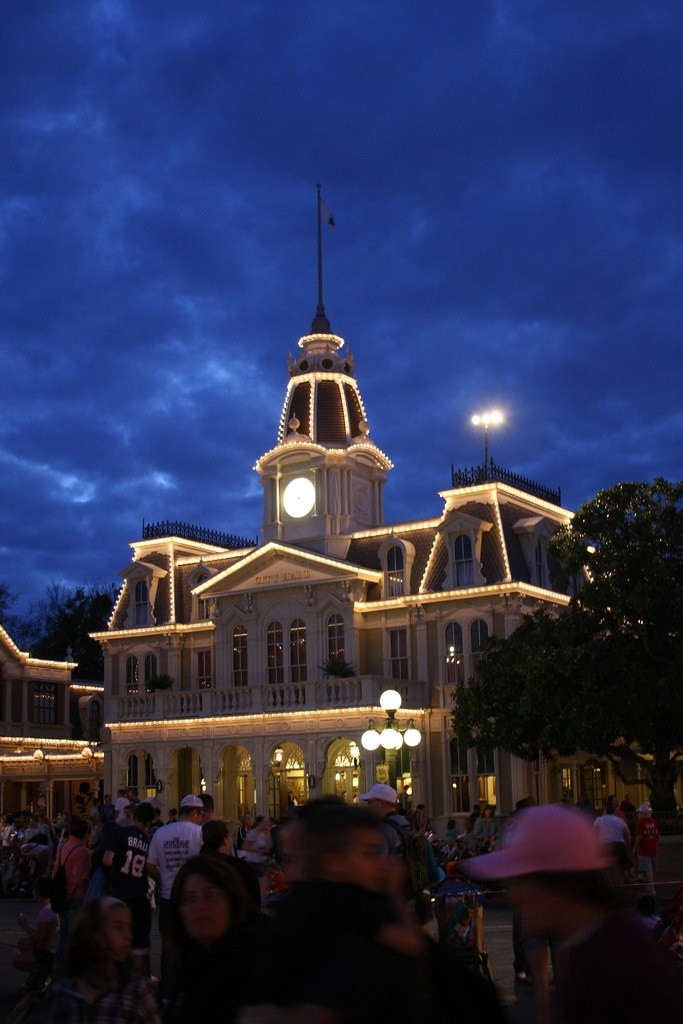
[361,690,422,792]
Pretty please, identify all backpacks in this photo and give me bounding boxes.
[47,845,80,913]
[383,817,440,885]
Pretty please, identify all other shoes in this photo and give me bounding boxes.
[646,886,655,896]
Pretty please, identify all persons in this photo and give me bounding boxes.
[0,786,683,1024]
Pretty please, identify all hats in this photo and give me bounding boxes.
[636,805,648,814]
[180,795,203,807]
[361,784,397,803]
[466,803,609,878]
